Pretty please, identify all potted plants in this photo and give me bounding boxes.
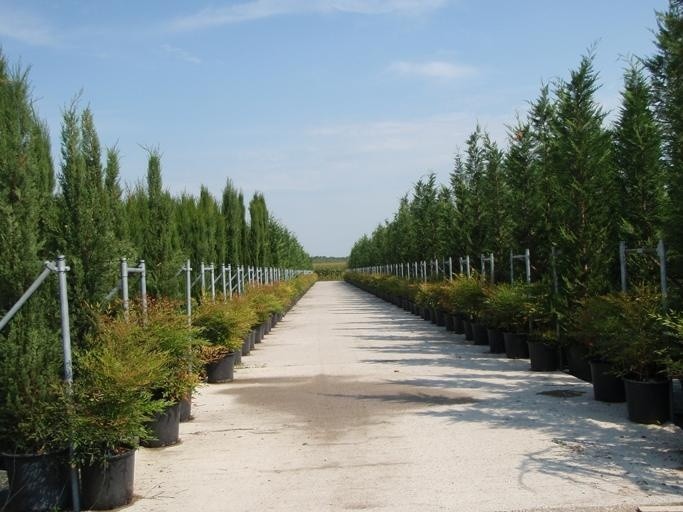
[182,271,317,384]
[342,270,495,345]
[1,294,212,511]
[488,269,682,427]
